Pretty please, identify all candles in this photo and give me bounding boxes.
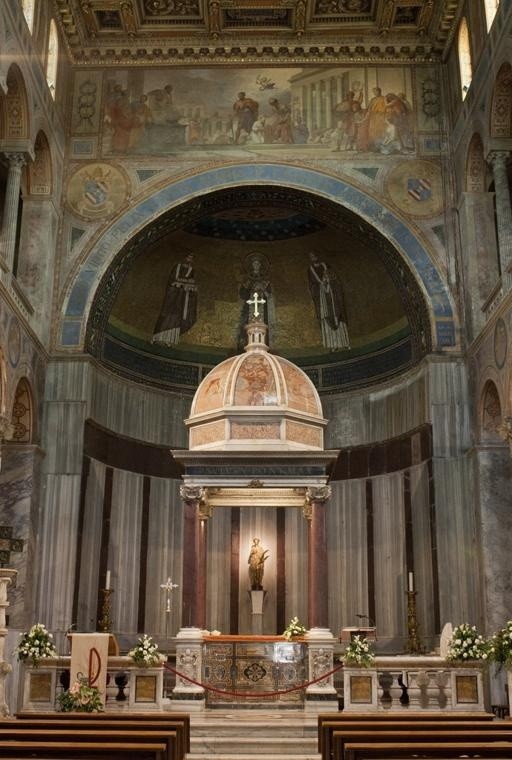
[105,570,111,590]
[408,571,414,592]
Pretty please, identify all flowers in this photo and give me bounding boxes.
[56,672,104,713]
[127,634,168,668]
[281,616,306,641]
[339,635,377,669]
[11,621,56,670]
[445,620,512,678]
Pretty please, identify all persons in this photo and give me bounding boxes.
[307,248,350,353]
[237,248,272,349]
[150,251,199,348]
[248,537,269,590]
